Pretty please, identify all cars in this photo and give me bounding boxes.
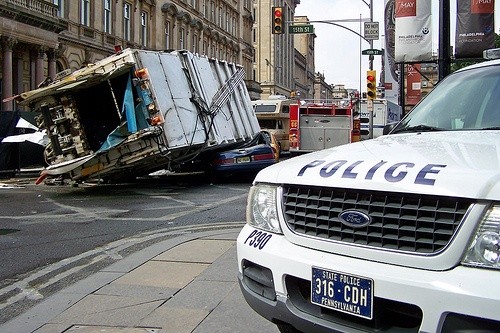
[203,129,282,184]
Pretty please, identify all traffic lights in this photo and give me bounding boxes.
[272,6,285,34]
[366,70,377,100]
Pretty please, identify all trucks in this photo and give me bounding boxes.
[249,95,296,153]
[359,99,403,142]
[0,43,262,186]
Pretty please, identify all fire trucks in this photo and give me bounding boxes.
[288,91,362,158]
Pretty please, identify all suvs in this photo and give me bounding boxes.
[236,47,500,333]
